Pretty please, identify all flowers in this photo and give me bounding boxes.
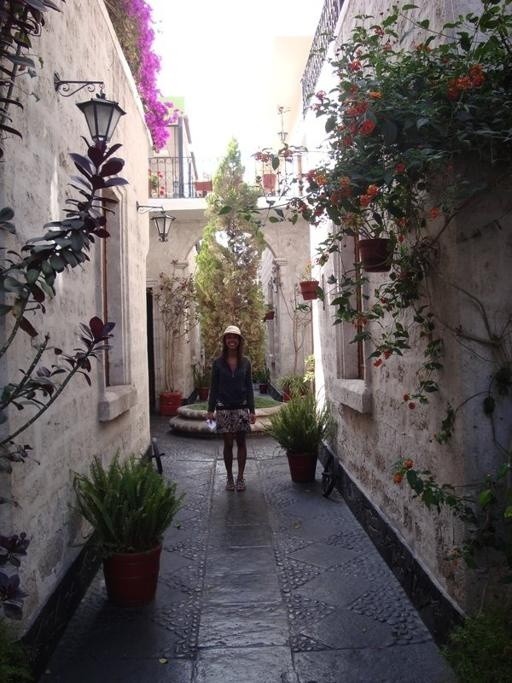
[302,259,315,281]
[148,270,200,392]
[341,180,404,238]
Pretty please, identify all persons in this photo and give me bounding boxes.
[206,324,257,492]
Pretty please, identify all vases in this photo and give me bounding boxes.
[299,280,320,299]
[355,238,396,271]
[160,390,182,415]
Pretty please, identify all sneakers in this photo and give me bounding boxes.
[236,478,246,491]
[226,480,234,491]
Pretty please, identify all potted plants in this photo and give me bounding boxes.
[262,304,274,321]
[68,445,176,608]
[192,364,211,401]
[255,369,272,394]
[276,374,308,403]
[256,389,336,488]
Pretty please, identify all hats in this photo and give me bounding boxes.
[219,326,245,343]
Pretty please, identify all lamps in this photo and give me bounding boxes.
[136,202,176,242]
[51,70,127,151]
